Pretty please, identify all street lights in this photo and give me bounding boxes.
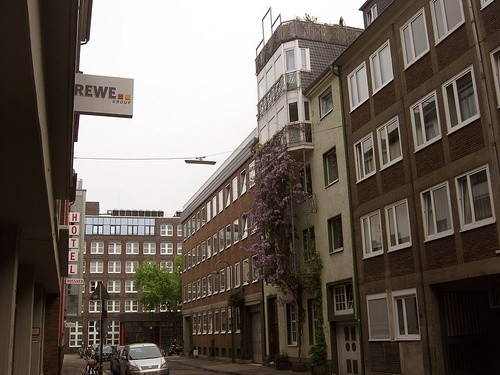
[211,260,236,364]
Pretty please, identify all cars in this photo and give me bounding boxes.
[111,342,170,375]
[86,343,114,362]
